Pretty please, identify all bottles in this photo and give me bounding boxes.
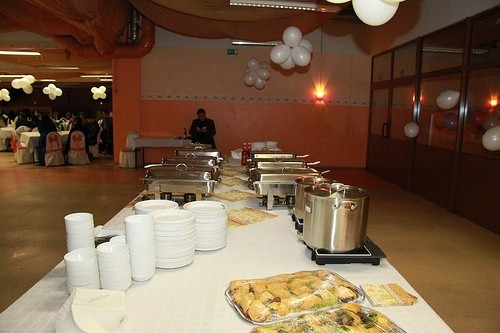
[184,128,187,140]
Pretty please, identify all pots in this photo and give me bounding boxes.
[294,177,339,219]
[302,183,369,252]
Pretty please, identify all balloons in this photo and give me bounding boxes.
[435,90,460,110]
[482,127,500,151]
[11,75,36,94]
[403,122,420,138]
[42,83,63,100]
[352,0,400,26]
[270,26,313,70]
[91,85,107,100]
[0,89,11,102]
[243,58,272,89]
[433,106,500,130]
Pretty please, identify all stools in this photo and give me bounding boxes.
[118,150,142,168]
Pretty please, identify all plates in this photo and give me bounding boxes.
[149,209,196,268]
[183,201,228,251]
[135,200,179,215]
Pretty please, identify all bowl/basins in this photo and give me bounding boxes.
[125,216,156,282]
[64,248,100,295]
[110,235,125,243]
[64,213,95,252]
[97,242,132,292]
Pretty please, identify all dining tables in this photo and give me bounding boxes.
[0,156,454,333]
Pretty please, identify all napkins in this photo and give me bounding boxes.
[53,286,131,333]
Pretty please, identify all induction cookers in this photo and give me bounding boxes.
[291,213,304,233]
[303,236,386,266]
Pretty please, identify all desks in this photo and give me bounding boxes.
[126,139,192,169]
[21,131,70,165]
[0,126,15,151]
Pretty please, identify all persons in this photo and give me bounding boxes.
[188,108,216,149]
[0,108,112,166]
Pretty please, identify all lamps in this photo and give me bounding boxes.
[230,0,341,12]
[315,94,324,103]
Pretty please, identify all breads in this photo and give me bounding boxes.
[230,269,392,333]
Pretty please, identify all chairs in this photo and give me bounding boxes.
[45,132,63,167]
[12,129,33,163]
[68,131,89,165]
[88,131,101,160]
